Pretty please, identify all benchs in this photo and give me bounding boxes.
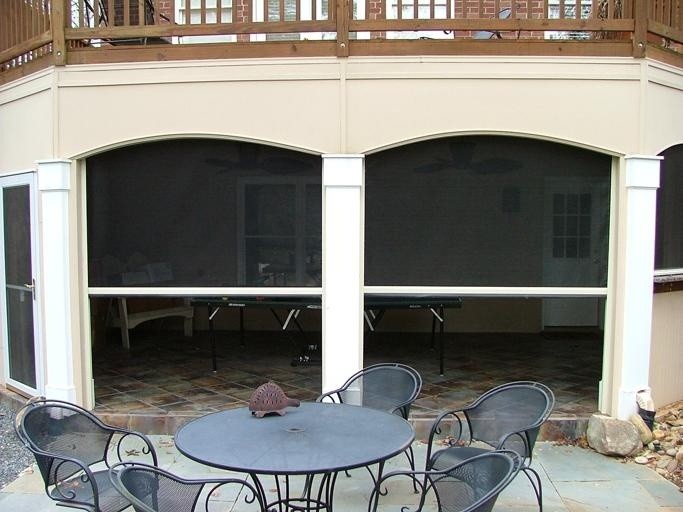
[103,268,194,352]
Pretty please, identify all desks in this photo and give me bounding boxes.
[188,296,462,376]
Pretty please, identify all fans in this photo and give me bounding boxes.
[410,137,522,180]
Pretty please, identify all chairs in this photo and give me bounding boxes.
[300,363,423,499]
[82,1,182,48]
[368,450,522,512]
[426,381,556,512]
[14,400,158,512]
[414,3,522,41]
[110,462,264,512]
